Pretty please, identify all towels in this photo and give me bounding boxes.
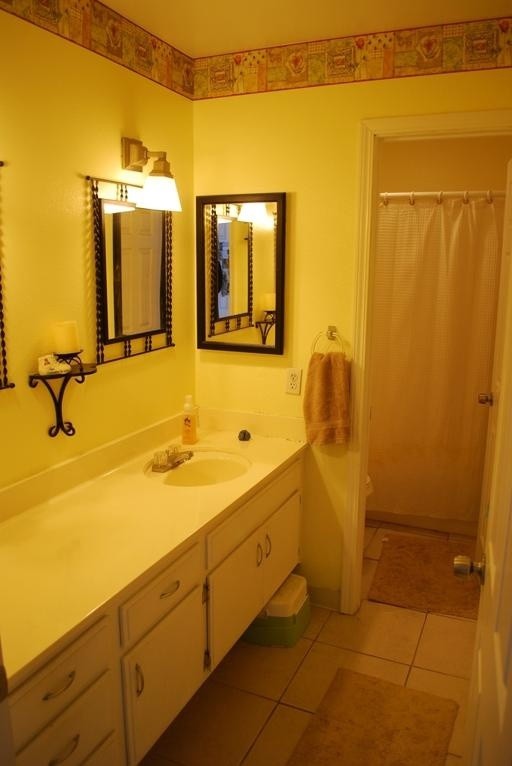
[304,351,354,445]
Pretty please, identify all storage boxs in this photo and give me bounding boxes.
[239,574,311,648]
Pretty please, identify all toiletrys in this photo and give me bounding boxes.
[180,392,198,446]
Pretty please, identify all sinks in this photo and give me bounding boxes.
[142,446,254,488]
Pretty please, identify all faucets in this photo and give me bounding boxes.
[151,442,193,474]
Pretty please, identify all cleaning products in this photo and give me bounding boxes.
[181,395,198,445]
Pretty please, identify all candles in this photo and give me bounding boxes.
[263,292,275,310]
[51,319,80,354]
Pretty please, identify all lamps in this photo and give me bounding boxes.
[216,218,232,224]
[120,134,183,213]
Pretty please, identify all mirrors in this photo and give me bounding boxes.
[206,203,255,337]
[103,201,136,214]
[85,174,286,365]
[235,202,268,222]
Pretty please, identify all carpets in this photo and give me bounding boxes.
[286,667,460,765]
[367,532,481,621]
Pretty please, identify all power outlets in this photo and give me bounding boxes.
[284,366,302,395]
